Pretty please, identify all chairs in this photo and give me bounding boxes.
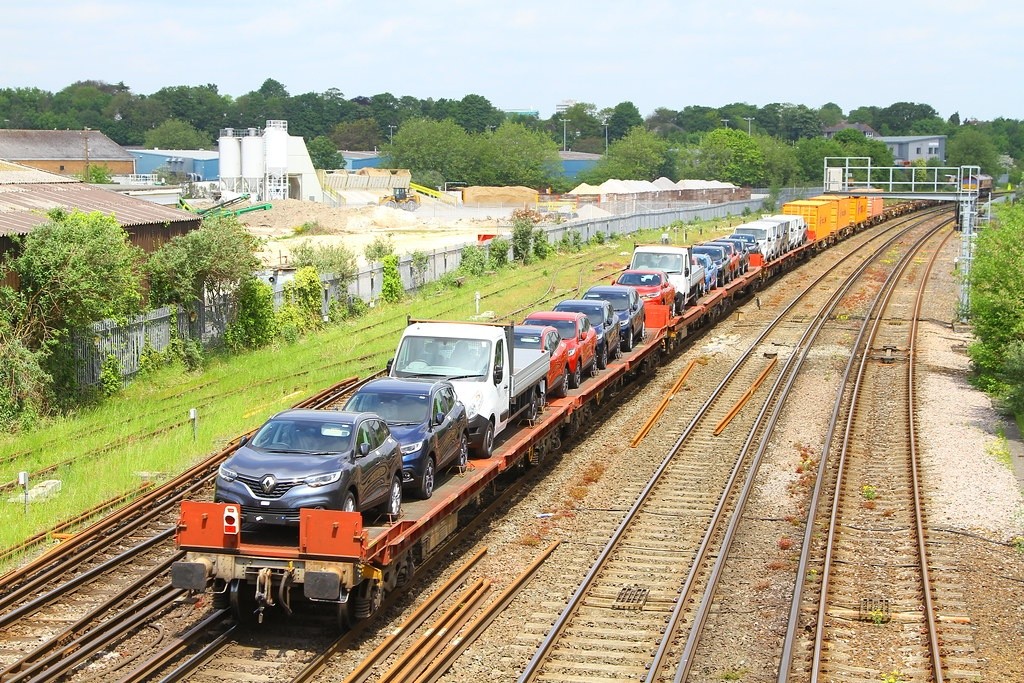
[659,257,671,268]
[446,342,475,369]
[652,275,659,284]
[419,342,441,366]
[672,257,682,270]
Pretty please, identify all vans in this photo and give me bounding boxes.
[733,215,807,263]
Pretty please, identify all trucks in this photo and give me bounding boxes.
[626,241,705,316]
[385,314,550,459]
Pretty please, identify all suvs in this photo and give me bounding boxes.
[339,376,470,501]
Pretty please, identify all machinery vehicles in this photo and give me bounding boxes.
[379,186,421,212]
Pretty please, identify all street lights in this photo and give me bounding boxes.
[559,119,571,151]
[743,118,755,137]
[721,119,730,129]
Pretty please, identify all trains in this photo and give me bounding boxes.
[171,172,994,635]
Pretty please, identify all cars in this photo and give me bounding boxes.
[520,310,598,389]
[579,285,644,352]
[611,269,676,321]
[691,234,761,292]
[214,408,404,524]
[551,299,620,371]
[512,326,570,412]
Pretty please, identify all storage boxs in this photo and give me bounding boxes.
[782,188,884,242]
